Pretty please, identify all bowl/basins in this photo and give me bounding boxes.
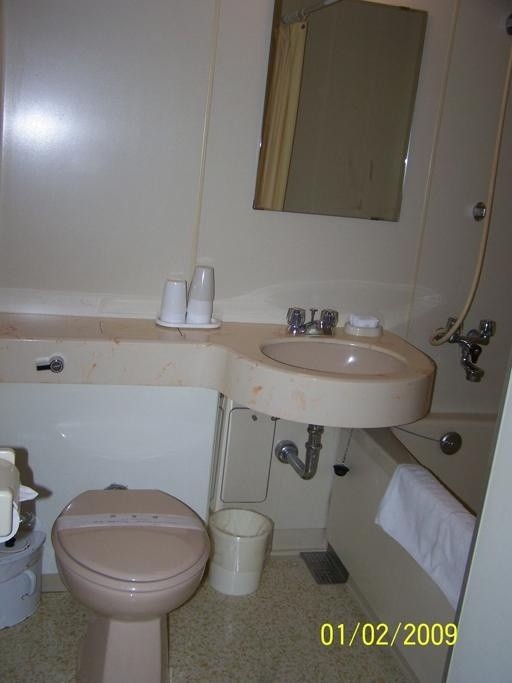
[343,321,384,339]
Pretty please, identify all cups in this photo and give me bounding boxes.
[160,263,215,325]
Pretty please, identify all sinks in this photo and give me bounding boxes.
[260,336,409,375]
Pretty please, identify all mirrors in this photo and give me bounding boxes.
[249,0,429,222]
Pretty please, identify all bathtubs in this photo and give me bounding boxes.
[323,413,499,683]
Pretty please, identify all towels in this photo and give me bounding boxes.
[371,461,479,612]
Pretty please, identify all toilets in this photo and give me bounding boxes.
[50,488,211,683]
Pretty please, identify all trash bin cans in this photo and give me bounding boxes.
[207,508,272,597]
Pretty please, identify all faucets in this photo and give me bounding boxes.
[303,320,329,335]
